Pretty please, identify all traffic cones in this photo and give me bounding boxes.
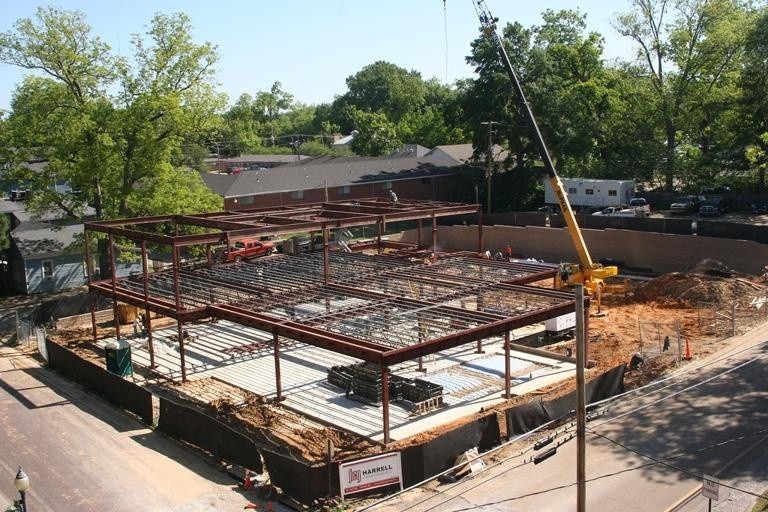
[683,336,694,360]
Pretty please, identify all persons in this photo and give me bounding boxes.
[504,243,511,261]
[485,248,492,259]
[388,189,399,208]
[494,251,502,260]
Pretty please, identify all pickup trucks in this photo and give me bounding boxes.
[215,239,276,263]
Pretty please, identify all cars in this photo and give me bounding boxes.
[276,234,324,255]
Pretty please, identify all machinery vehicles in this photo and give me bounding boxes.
[466,0,632,313]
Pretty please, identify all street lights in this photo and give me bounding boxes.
[478,118,505,216]
[7,465,30,511]
